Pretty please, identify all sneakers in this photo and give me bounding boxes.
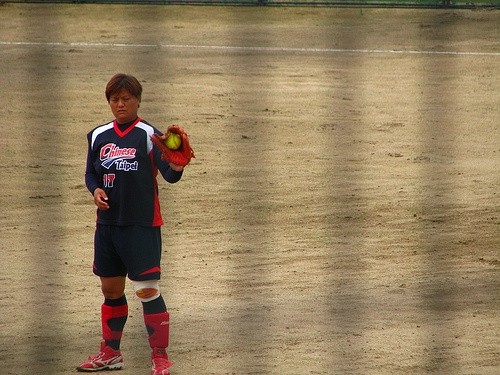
[76,341,124,371]
[149,352,173,375]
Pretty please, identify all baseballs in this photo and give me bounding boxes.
[165,132,182,150]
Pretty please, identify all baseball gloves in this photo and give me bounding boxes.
[150,124,193,166]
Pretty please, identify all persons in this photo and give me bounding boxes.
[77,74,196,374]
